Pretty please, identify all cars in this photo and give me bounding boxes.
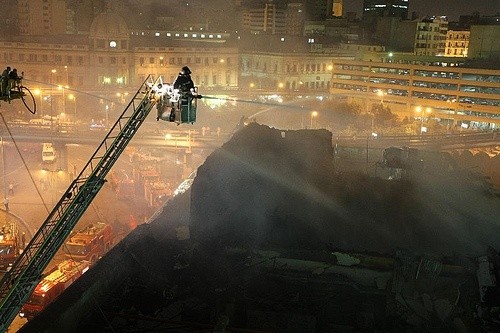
[6,108,211,232]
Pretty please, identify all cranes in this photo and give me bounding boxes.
[0,73,199,333]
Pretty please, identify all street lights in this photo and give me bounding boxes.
[67,92,78,124]
[309,110,318,128]
[35,89,44,117]
[415,106,432,135]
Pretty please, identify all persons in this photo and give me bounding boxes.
[0,66,11,89]
[174,66,196,103]
[5,69,22,99]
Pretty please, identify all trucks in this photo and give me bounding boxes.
[1,222,20,268]
[19,256,91,323]
[63,222,117,264]
